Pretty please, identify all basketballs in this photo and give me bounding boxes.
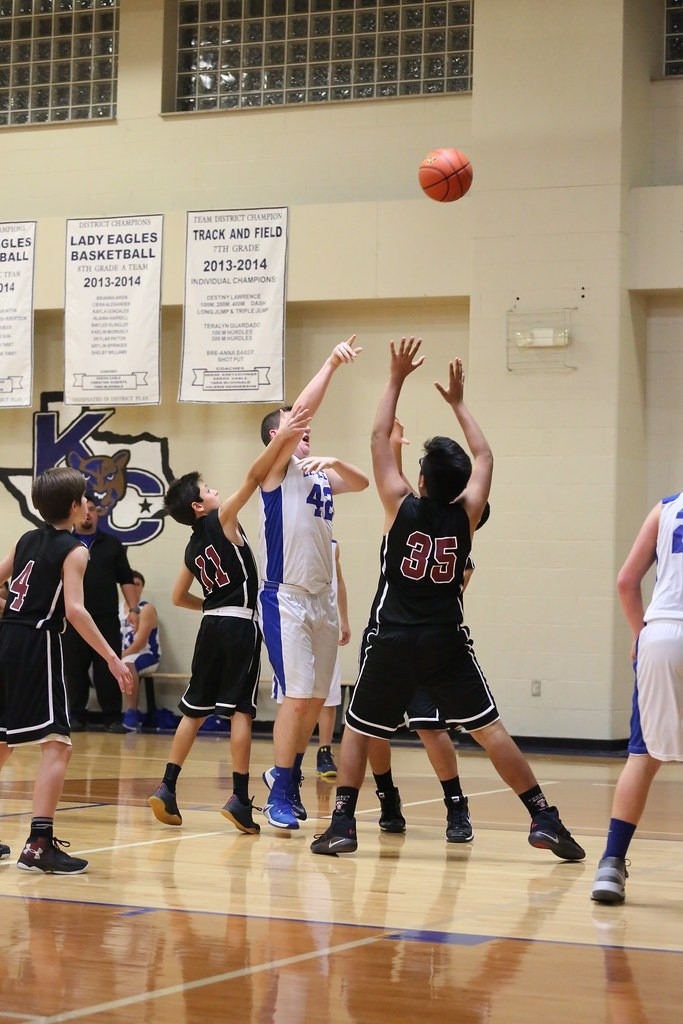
[418,146,474,204]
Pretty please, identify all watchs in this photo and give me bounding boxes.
[129,607,141,614]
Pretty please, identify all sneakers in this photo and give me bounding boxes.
[591,856,632,902]
[147,783,182,826]
[376,785,406,833]
[263,793,299,829]
[102,720,126,732]
[528,805,586,860]
[0,844,10,860]
[310,812,358,853]
[315,746,337,776]
[263,766,308,821]
[17,837,88,875]
[443,794,474,843]
[220,793,262,834]
[121,709,143,730]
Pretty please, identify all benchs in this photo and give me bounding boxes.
[140,673,356,740]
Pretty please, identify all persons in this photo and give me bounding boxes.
[256,333,370,829]
[367,418,491,844]
[0,581,9,619]
[0,467,134,875]
[590,492,683,902]
[147,405,312,834]
[310,336,586,860]
[269,539,352,777]
[67,496,140,734]
[88,571,161,730]
